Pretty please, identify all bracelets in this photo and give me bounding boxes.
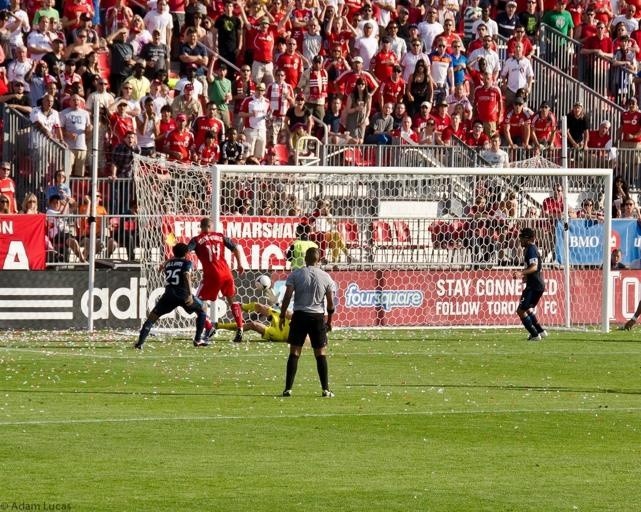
[632,316,638,322]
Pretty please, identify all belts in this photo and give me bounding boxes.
[260,61,269,63]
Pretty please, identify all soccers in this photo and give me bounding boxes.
[255,274,271,291]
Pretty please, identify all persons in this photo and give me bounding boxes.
[277,245,339,398]
[623,300,640,330]
[1,1,641,275]
[133,239,206,351]
[156,216,246,343]
[516,225,550,342]
[209,288,302,347]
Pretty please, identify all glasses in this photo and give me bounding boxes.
[357,83,364,85]
[627,102,636,106]
[99,82,107,85]
[625,203,632,206]
[514,102,522,106]
[426,123,433,126]
[412,44,418,46]
[28,200,35,203]
[540,105,548,108]
[439,45,444,48]
[417,65,424,67]
[597,26,604,29]
[296,97,305,102]
[616,179,623,183]
[0,199,8,203]
[516,31,523,33]
[454,46,459,48]
[587,13,594,16]
[394,70,401,73]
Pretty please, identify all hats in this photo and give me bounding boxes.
[313,55,323,63]
[407,24,417,30]
[260,19,269,24]
[438,100,448,107]
[176,113,185,121]
[65,59,74,65]
[184,83,193,90]
[394,66,401,72]
[150,78,161,85]
[218,63,225,69]
[353,56,362,63]
[400,8,408,15]
[382,36,390,43]
[420,100,430,109]
[117,99,127,106]
[79,12,90,20]
[506,1,517,7]
[513,97,524,104]
[255,82,265,90]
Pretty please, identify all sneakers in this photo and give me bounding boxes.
[282,389,292,396]
[193,339,209,347]
[234,330,242,342]
[321,389,336,397]
[538,330,548,337]
[527,333,541,341]
[203,327,216,340]
